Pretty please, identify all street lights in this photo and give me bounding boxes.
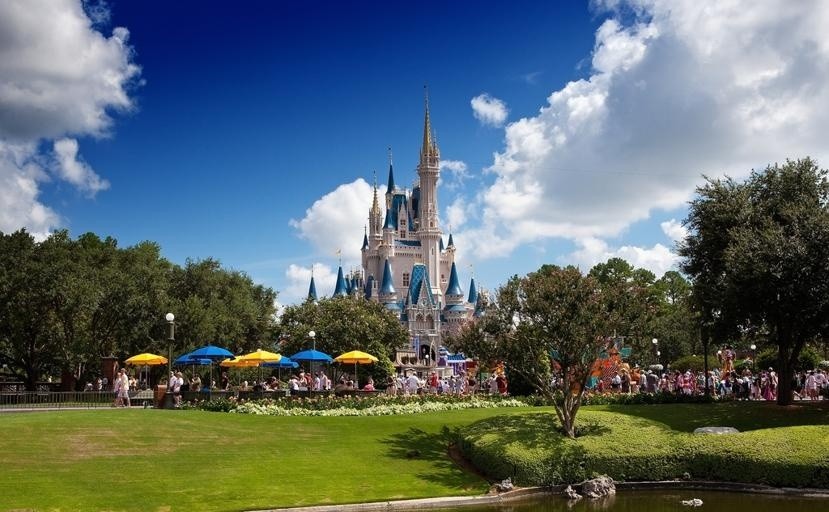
[656,351,661,364]
[652,337,657,365]
[424,354,431,378]
[718,350,722,364]
[164,312,176,410]
[750,344,758,375]
[307,330,317,376]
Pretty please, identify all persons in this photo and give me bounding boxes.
[96,377,104,390]
[169,370,212,408]
[112,369,136,408]
[242,377,277,390]
[220,372,229,387]
[793,369,828,400]
[482,374,507,394]
[365,376,374,390]
[289,370,353,390]
[597,368,777,401]
[386,371,477,396]
[716,342,736,371]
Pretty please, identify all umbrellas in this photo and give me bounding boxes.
[336,348,381,387]
[262,355,301,382]
[187,342,235,387]
[174,350,213,379]
[288,348,333,396]
[240,348,281,396]
[219,355,260,390]
[123,351,168,389]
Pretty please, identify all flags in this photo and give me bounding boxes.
[336,247,341,256]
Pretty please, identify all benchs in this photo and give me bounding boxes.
[174,390,378,402]
